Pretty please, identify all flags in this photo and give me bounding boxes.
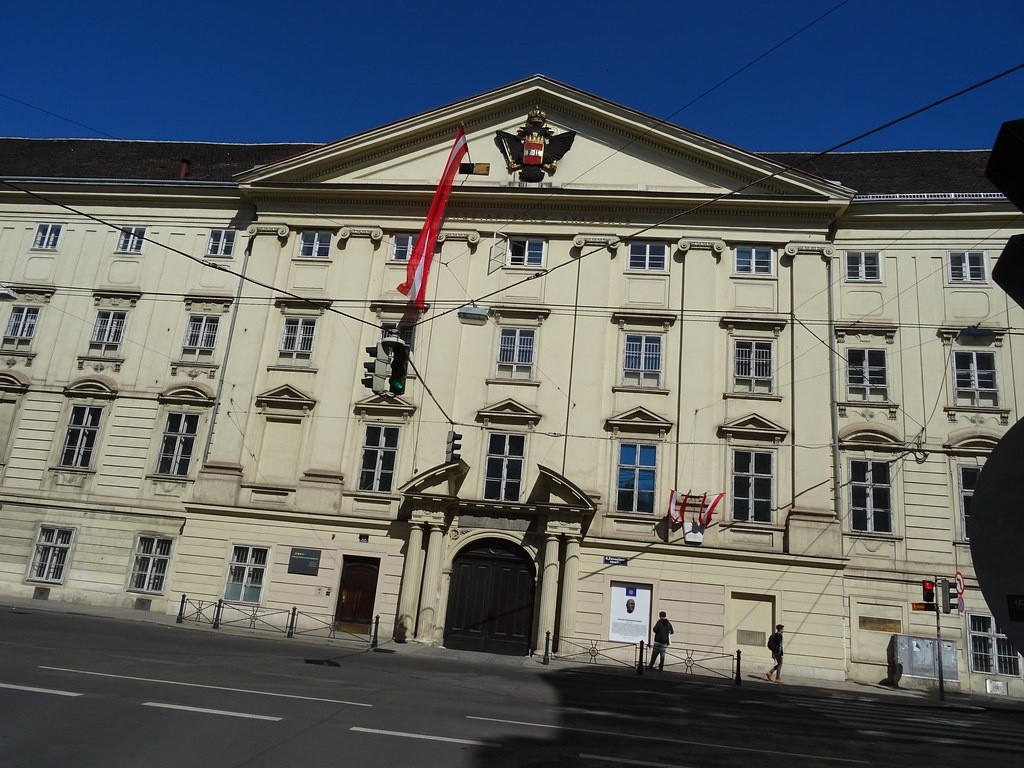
[699,493,724,525]
[396,126,468,310]
[669,491,690,522]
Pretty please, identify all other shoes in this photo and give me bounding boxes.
[657,669,663,672]
[645,667,652,672]
[775,678,782,683]
[766,671,772,681]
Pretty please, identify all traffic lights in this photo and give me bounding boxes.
[389,344,410,396]
[922,580,934,602]
[941,580,959,614]
[360,341,390,395]
[445,429,462,464]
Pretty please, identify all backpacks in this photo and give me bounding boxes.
[768,633,779,650]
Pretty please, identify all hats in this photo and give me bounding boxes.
[776,624,784,629]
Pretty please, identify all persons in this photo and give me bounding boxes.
[649,612,674,671]
[766,625,784,684]
